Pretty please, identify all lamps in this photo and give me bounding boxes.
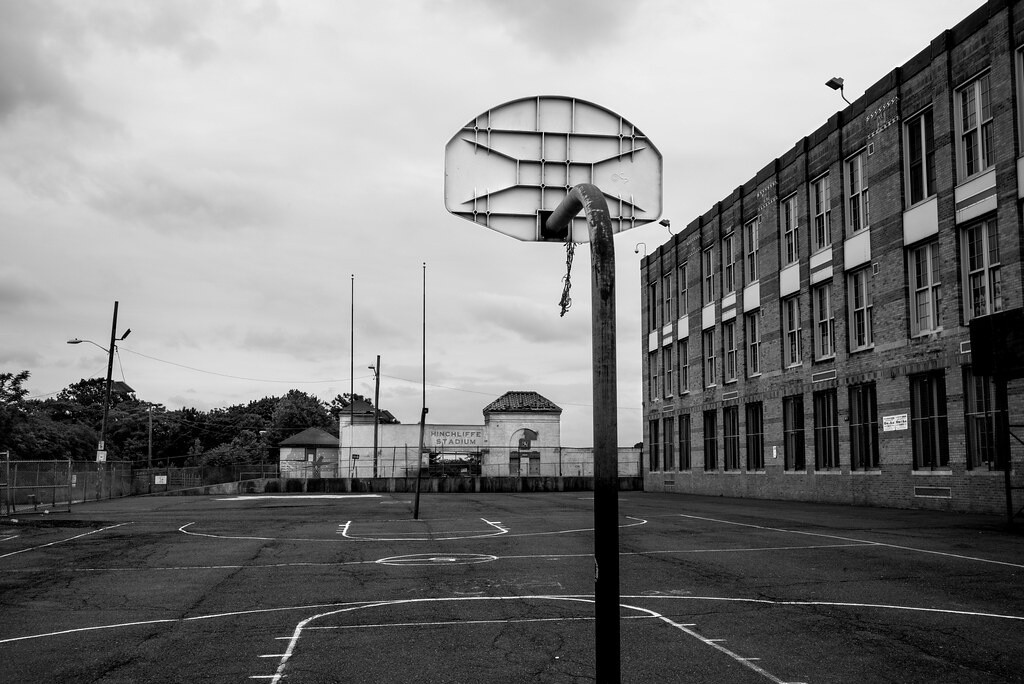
[825,77,851,105]
[635,242,646,257]
[659,219,673,236]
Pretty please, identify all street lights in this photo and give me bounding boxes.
[66,339,113,502]
[368,365,380,478]
